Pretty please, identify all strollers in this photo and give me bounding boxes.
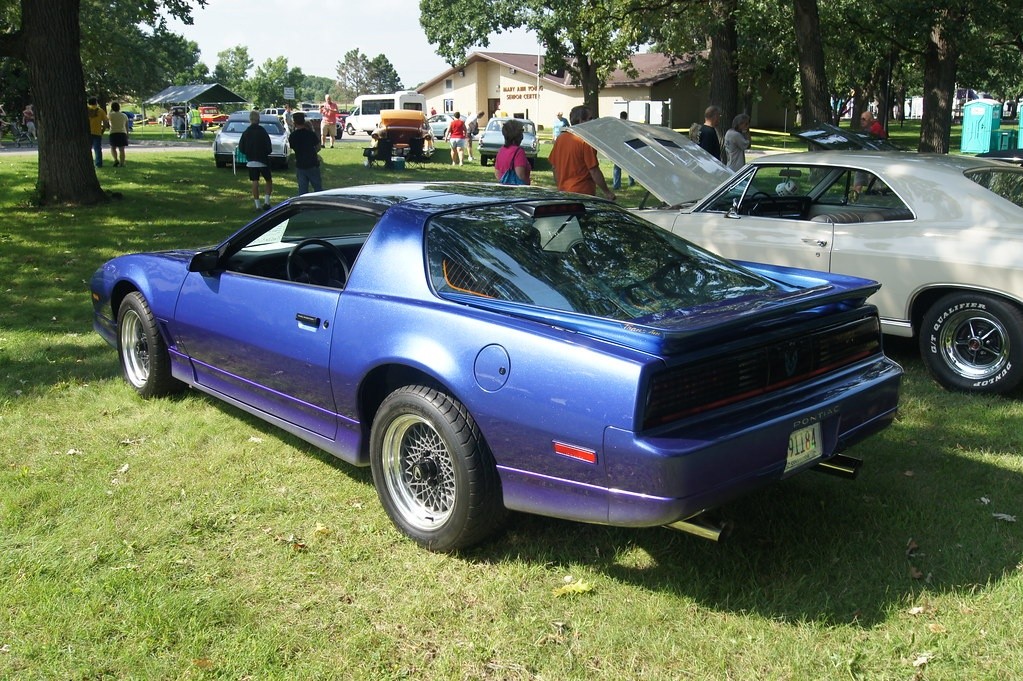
[9,121,34,149]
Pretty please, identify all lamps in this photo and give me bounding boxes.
[510,68,516,74]
[459,70,464,77]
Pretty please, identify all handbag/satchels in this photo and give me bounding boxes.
[499,168,528,187]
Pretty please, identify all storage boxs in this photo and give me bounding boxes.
[391,157,405,169]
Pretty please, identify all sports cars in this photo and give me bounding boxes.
[91,180,905,556]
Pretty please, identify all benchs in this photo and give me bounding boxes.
[174,129,192,138]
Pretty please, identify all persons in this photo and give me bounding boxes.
[464,111,484,161]
[88,97,109,168]
[238,111,272,211]
[725,114,751,172]
[445,112,467,166]
[22,106,37,140]
[319,95,339,148]
[495,120,531,185]
[107,102,129,167]
[548,105,617,200]
[283,104,315,131]
[493,108,508,128]
[689,106,721,161]
[553,112,570,141]
[612,111,634,189]
[854,112,886,197]
[0,107,11,149]
[172,104,204,140]
[431,107,437,119]
[288,114,322,195]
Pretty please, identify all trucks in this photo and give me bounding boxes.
[344,90,427,136]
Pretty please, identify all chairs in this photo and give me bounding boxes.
[232,145,248,175]
[863,210,914,221]
[811,213,863,223]
[405,137,426,169]
[364,138,393,168]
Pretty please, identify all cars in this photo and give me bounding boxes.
[260,102,350,130]
[212,114,291,170]
[158,113,169,124]
[370,108,437,161]
[785,121,1023,211]
[290,110,344,140]
[477,117,540,170]
[564,114,1023,401]
[427,113,468,140]
[133,113,142,121]
[164,106,189,127]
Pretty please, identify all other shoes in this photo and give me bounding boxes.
[320,145,325,148]
[330,145,335,148]
[112,160,120,167]
[263,204,271,210]
[458,162,464,166]
[256,208,264,211]
[467,157,476,162]
[452,162,456,166]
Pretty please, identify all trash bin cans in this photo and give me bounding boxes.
[997,128,1018,151]
[122,111,135,129]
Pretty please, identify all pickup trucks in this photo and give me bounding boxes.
[199,106,229,128]
